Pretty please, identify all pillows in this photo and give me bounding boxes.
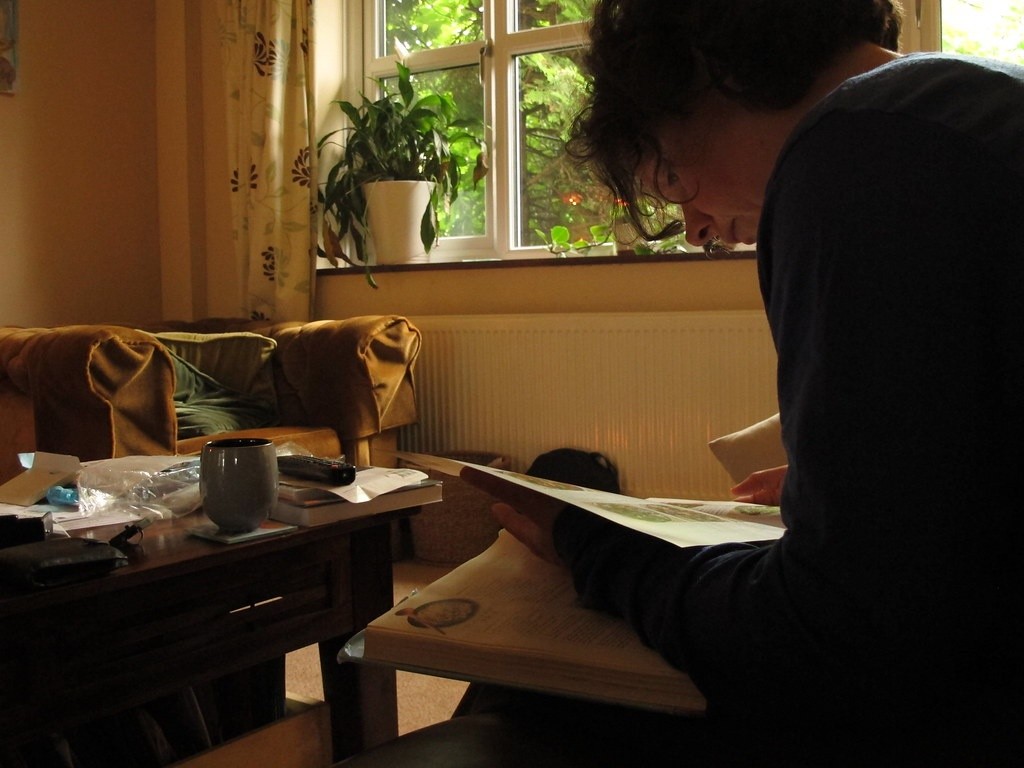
[710,412,790,482]
[167,348,275,440]
[135,327,281,427]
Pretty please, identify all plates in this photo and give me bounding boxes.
[185,522,298,544]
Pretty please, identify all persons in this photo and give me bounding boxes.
[459,0,1024,768]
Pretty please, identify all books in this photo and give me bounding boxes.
[272,478,445,527]
[337,449,789,714]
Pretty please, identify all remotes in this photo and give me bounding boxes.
[277,455,357,486]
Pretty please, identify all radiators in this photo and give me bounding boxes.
[398,311,779,501]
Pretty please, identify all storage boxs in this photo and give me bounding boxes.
[161,692,332,768]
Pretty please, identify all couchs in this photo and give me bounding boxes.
[0,315,422,471]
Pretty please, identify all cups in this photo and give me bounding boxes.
[199,438,278,534]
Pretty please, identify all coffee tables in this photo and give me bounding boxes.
[0,505,424,760]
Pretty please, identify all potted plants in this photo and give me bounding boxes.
[535,200,688,256]
[317,61,490,288]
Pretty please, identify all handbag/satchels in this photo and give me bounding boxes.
[526,447,621,494]
